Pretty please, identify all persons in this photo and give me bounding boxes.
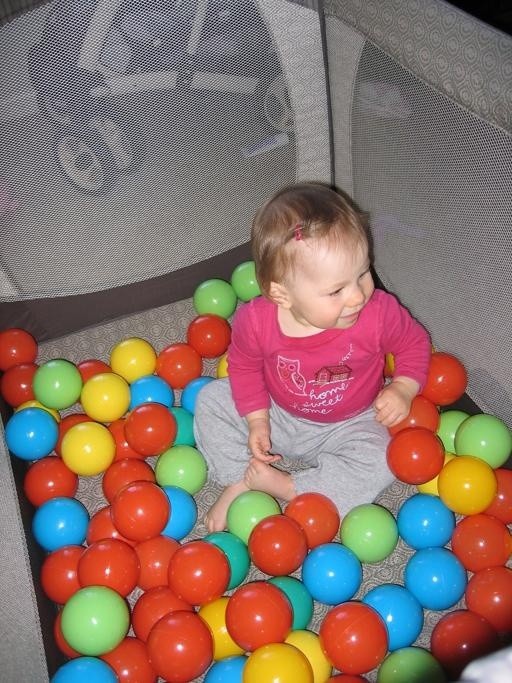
[190,180,432,536]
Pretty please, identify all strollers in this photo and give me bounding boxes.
[30,0,295,193]
